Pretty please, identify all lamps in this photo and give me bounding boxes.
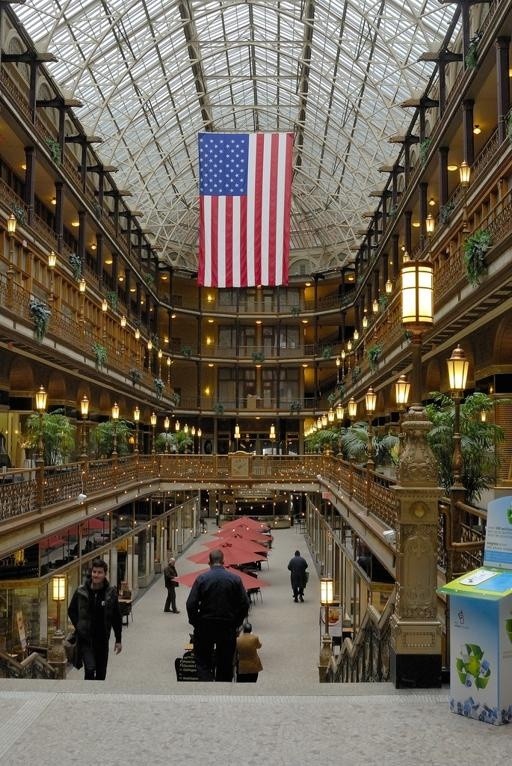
[445,342,470,400]
[205,387,209,396]
[303,365,308,367]
[301,396,358,439]
[7,164,176,368]
[208,294,212,304]
[208,319,214,324]
[28,387,202,446]
[366,385,376,421]
[256,320,262,325]
[51,573,67,635]
[208,363,213,366]
[335,121,486,365]
[208,337,211,347]
[303,320,309,323]
[319,578,334,639]
[234,425,241,438]
[305,282,311,286]
[270,425,276,439]
[394,373,411,412]
[255,365,260,367]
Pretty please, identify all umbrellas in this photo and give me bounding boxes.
[176,516,272,593]
[40,518,109,549]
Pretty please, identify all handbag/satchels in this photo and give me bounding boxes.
[63,632,83,669]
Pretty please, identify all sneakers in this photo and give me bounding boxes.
[295,596,304,602]
[164,609,180,613]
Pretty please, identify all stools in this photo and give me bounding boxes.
[249,590,264,607]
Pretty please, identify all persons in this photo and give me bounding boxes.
[68,559,122,680]
[186,550,250,682]
[236,623,263,682]
[165,558,180,613]
[242,568,257,604]
[288,551,308,602]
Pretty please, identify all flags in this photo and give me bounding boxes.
[197,133,294,287]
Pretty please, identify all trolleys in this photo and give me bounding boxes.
[174,624,243,682]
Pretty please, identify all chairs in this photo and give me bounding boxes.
[26,645,48,659]
[119,590,134,628]
